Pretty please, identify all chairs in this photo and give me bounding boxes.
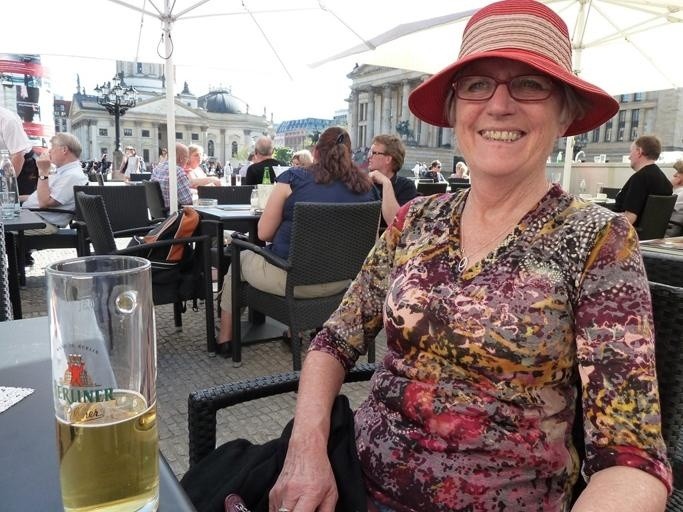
[73,184,166,256]
[416,177,470,194]
[196,186,256,321]
[78,194,215,356]
[634,194,676,240]
[231,201,381,398]
[642,256,683,287]
[143,182,168,219]
[17,185,81,290]
[188,254,681,512]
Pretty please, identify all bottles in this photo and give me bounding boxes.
[224,160,232,185]
[557,151,562,163]
[0,149,20,216]
[262,167,272,184]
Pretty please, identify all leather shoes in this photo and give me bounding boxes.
[215,340,232,359]
[283,330,302,348]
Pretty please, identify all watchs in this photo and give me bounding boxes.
[38,174,49,181]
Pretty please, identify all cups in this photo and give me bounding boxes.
[45,254,160,512]
[596,193,608,199]
[258,185,273,209]
[594,155,600,163]
[236,175,241,185]
[600,154,607,165]
[0,192,16,220]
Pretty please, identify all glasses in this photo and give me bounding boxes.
[368,150,385,157]
[451,75,563,102]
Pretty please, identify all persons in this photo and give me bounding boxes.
[246,137,283,184]
[449,161,466,180]
[425,160,449,187]
[666,159,683,236]
[291,149,313,167]
[120,150,141,178]
[367,134,416,236]
[6,131,88,236]
[1,103,40,205]
[151,141,194,211]
[214,127,381,361]
[120,147,131,177]
[612,136,673,237]
[199,159,243,181]
[238,151,259,178]
[185,144,221,202]
[269,1,672,512]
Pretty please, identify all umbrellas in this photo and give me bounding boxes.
[342,1,682,192]
[0,0,402,215]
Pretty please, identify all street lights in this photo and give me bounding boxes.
[94,72,139,151]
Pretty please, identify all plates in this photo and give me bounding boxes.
[594,199,609,202]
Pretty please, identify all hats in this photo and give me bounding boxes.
[409,1,620,137]
